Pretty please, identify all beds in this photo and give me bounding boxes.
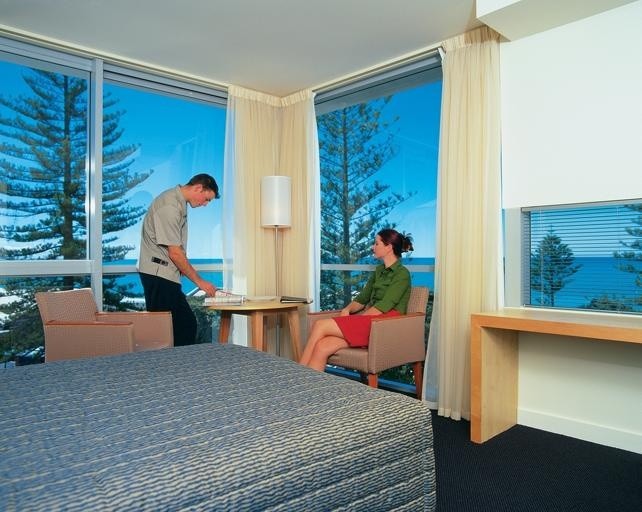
[0,343,437,512]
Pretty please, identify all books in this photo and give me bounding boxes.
[201,290,313,307]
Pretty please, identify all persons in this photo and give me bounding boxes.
[299,230,414,371]
[137,173,220,347]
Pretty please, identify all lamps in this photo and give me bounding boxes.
[260,175,292,357]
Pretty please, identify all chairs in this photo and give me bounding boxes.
[307,287,429,401]
[34,288,174,363]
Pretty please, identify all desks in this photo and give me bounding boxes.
[207,295,311,363]
